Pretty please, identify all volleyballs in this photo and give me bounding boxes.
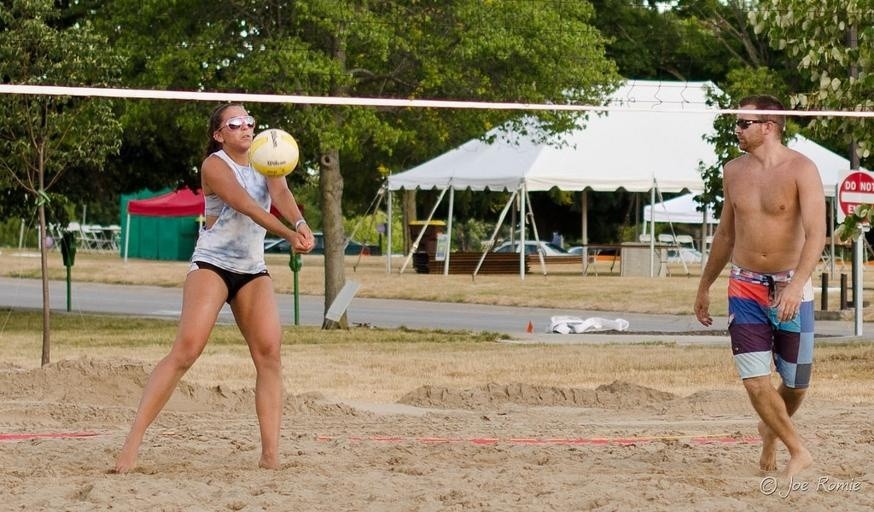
[248,129,300,178]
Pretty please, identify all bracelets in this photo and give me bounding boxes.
[296,220,306,230]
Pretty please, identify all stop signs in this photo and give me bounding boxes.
[837,172,874,224]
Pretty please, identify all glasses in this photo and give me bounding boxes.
[737,118,777,129]
[217,116,256,132]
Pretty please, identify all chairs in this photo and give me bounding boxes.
[46,221,121,256]
[639,233,702,275]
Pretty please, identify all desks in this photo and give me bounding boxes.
[569,241,679,279]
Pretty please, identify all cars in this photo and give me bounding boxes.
[263,233,371,256]
[493,239,577,257]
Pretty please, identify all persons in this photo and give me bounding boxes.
[115,104,315,474]
[694,95,827,477]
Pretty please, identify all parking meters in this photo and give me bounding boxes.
[288,245,303,326]
[60,232,75,310]
